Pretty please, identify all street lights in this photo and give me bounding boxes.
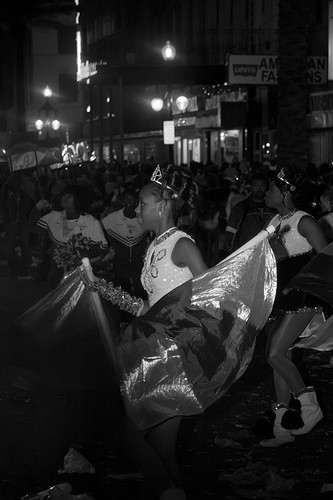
[149,40,190,168]
[35,82,62,148]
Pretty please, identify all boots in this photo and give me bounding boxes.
[257,402,297,448]
[281,384,325,436]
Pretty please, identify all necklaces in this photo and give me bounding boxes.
[154,226,179,246]
[278,209,301,220]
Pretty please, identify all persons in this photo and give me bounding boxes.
[76,163,213,500]
[0,160,333,327]
[259,163,333,448]
